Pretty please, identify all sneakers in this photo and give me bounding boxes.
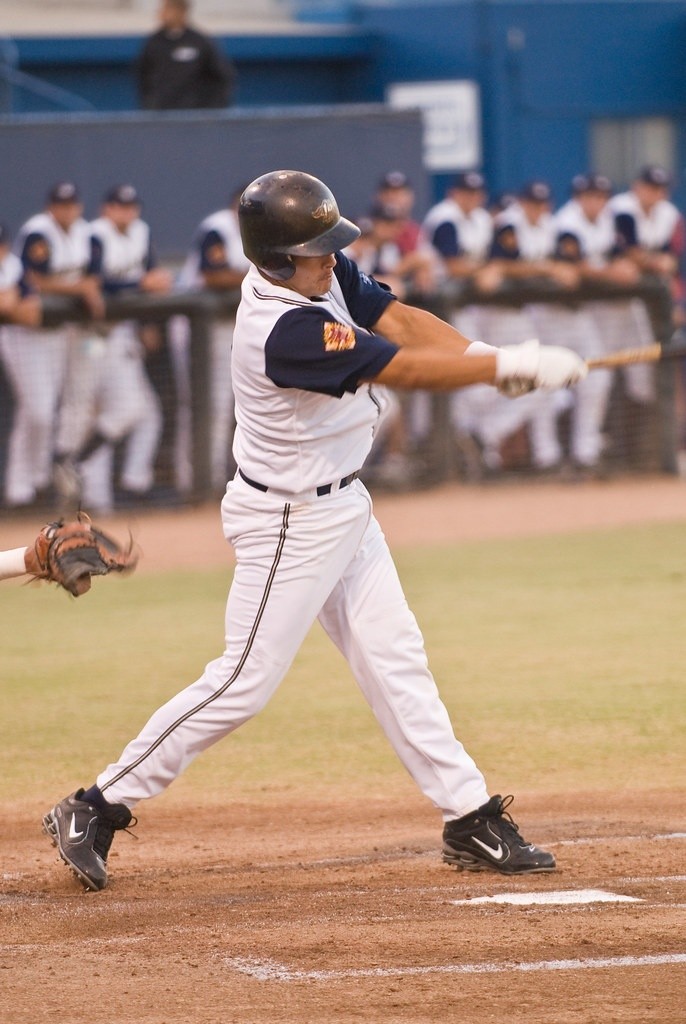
[440,794,558,875]
[41,787,131,891]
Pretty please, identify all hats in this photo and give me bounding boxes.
[577,174,611,195]
[105,184,144,206]
[456,170,487,192]
[641,164,672,188]
[378,170,414,190]
[523,180,552,203]
[47,180,83,203]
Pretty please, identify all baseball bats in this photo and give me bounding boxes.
[505,334,686,398]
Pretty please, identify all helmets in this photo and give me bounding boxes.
[237,169,364,281]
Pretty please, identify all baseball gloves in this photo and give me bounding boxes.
[20,498,141,599]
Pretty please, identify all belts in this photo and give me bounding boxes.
[239,467,362,496]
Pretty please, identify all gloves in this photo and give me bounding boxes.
[465,337,589,394]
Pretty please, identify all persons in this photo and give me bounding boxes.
[0,166,686,511]
[0,521,142,598]
[38,170,589,891]
[133,0,231,109]
